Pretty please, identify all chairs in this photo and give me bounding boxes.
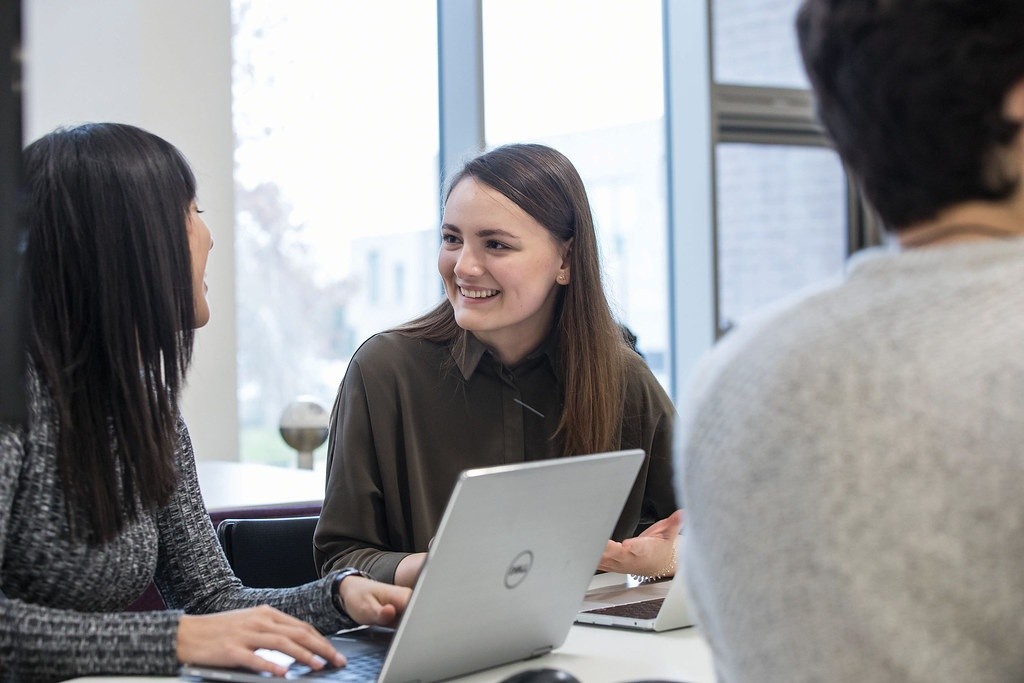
[218,514,319,590]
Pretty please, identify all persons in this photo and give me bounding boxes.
[677,0,1024,683]
[314,144,683,591]
[0,122,414,683]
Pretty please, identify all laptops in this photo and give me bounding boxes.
[573,575,697,632]
[179,448,645,683]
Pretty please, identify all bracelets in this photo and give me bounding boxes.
[632,541,675,582]
[331,570,375,621]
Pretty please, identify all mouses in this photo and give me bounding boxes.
[498,668,579,683]
